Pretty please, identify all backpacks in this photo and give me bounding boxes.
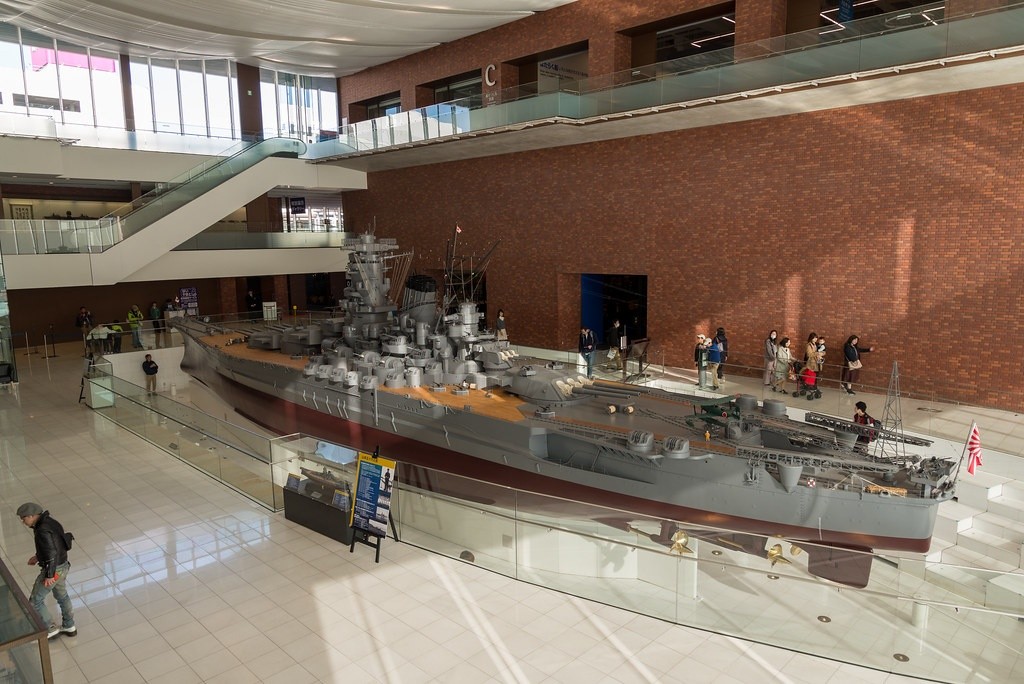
[856,414,881,442]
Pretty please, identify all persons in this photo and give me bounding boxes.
[495,310,508,337]
[127,305,143,348]
[854,402,875,453]
[77,306,123,353]
[695,327,728,390]
[150,299,173,332]
[763,330,799,394]
[384,469,390,492]
[65,211,73,220]
[17,502,77,639]
[607,320,623,370]
[842,335,874,395]
[143,354,158,396]
[806,333,826,392]
[246,289,258,324]
[578,326,598,378]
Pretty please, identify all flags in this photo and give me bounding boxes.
[968,428,983,475]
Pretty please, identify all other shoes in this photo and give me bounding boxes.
[711,386,719,390]
[780,389,788,394]
[617,368,623,370]
[695,383,699,385]
[59,625,77,637]
[31,629,61,644]
[841,383,847,391]
[846,388,855,395]
[148,393,151,397]
[772,387,776,392]
[607,366,615,369]
[152,392,158,396]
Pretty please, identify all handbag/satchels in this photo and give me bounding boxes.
[848,359,862,370]
[716,337,723,352]
[64,532,74,551]
[788,367,796,380]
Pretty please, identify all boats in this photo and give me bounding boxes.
[167,221,963,598]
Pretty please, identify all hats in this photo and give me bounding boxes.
[16,503,42,516]
[131,305,138,311]
[704,338,712,345]
[697,334,705,339]
